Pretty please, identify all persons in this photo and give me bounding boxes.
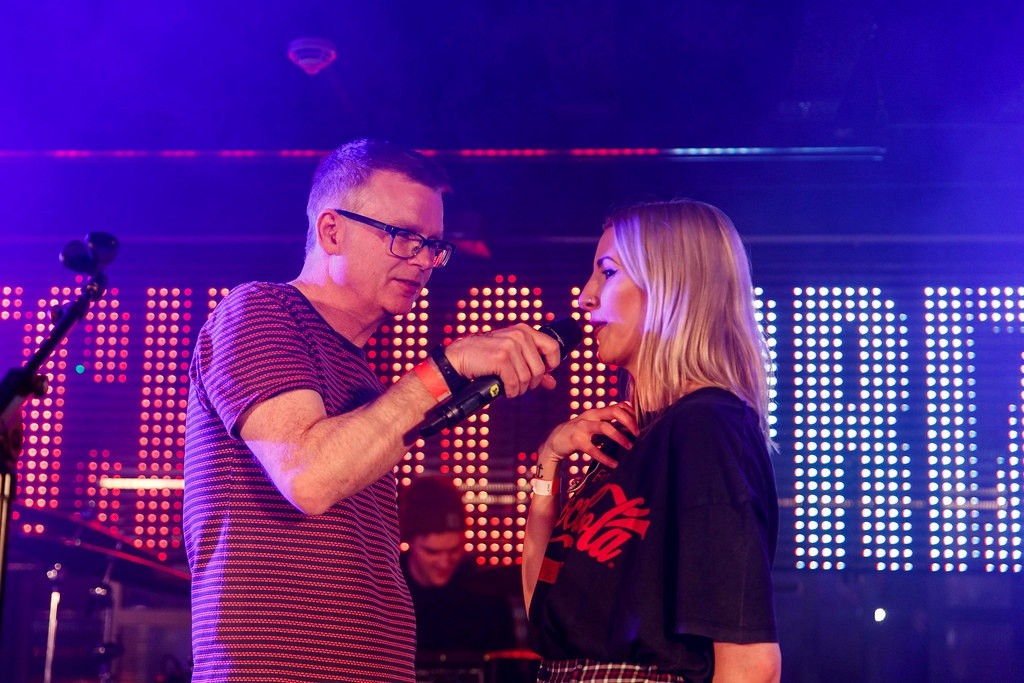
[181,138,560,682]
[398,473,517,683]
[522,200,781,683]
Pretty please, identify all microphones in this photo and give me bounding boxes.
[418,317,583,437]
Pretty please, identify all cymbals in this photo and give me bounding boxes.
[41,533,195,587]
[17,500,157,562]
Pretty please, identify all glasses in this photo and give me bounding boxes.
[334,209,456,269]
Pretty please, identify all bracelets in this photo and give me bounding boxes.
[414,343,470,404]
[530,478,560,496]
